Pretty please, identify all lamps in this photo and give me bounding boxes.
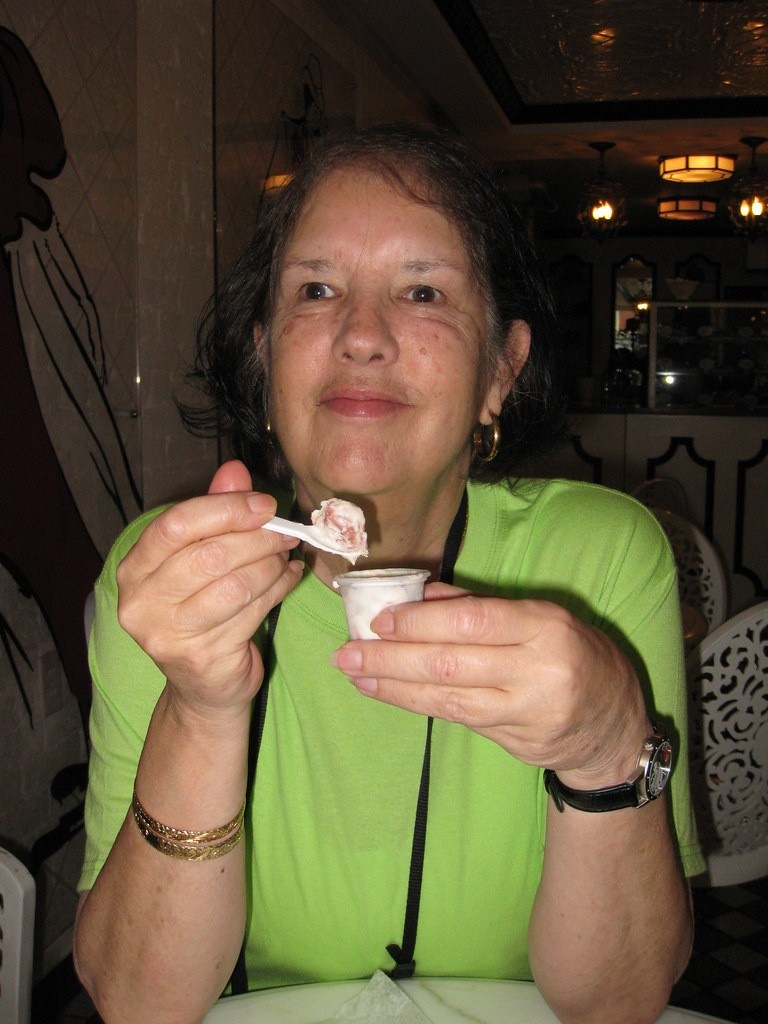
[655,152,737,184]
[653,195,718,221]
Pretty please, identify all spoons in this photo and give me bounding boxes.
[261,516,367,555]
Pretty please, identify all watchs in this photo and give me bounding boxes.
[543,726,673,813]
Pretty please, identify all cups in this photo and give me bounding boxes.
[333,567,431,641]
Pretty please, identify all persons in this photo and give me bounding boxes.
[68,108,706,1024]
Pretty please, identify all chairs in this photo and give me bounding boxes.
[630,477,768,888]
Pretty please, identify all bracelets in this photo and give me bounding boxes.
[131,777,247,862]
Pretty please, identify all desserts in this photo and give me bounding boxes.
[310,497,367,554]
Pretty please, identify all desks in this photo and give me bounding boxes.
[628,301,768,408]
